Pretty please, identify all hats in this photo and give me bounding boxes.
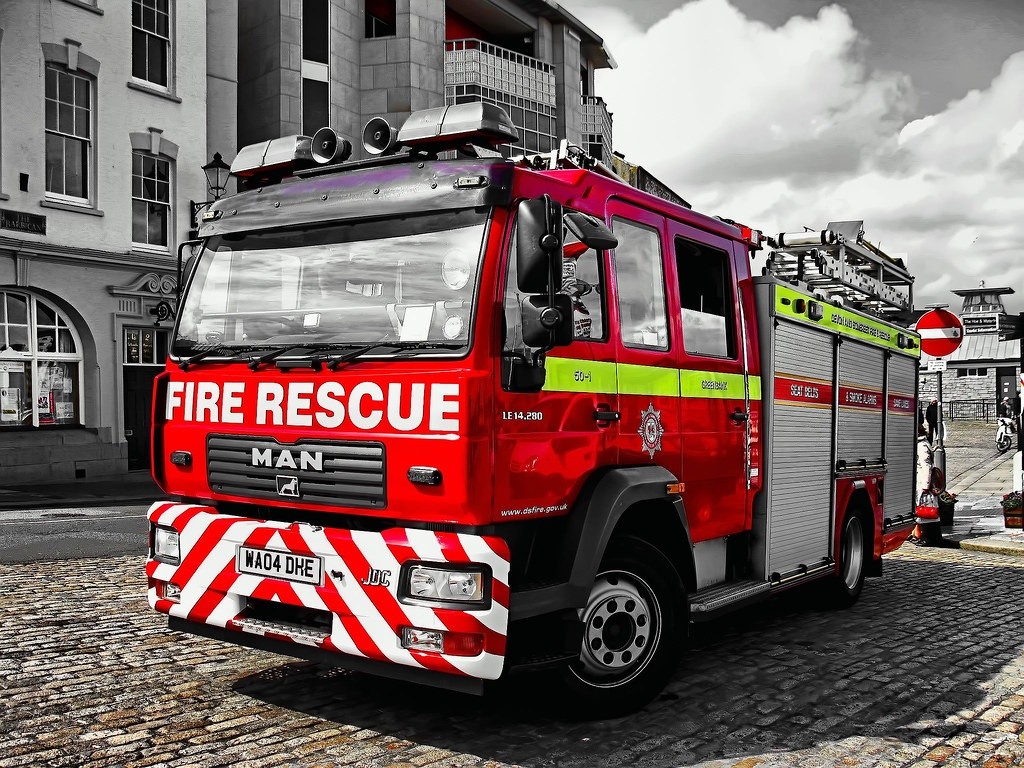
[931,397,937,403]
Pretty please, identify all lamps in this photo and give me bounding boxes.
[190,152,231,229]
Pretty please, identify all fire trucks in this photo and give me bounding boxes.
[145,98,922,718]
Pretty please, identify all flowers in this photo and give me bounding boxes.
[939,491,959,504]
[1001,491,1022,510]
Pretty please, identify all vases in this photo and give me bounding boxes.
[939,504,954,526]
[1004,506,1024,527]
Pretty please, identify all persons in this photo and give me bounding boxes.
[1000,389,1018,434]
[909,401,946,547]
[292,255,388,335]
[1013,388,1021,433]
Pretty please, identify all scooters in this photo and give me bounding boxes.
[995,415,1018,452]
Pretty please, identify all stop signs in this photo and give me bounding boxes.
[916,310,965,357]
